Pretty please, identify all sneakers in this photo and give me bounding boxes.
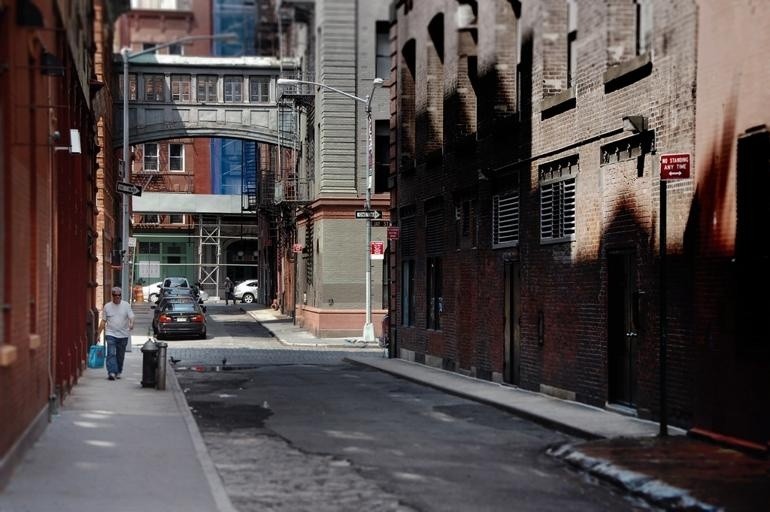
[109,373,121,381]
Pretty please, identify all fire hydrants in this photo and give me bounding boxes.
[141,338,158,388]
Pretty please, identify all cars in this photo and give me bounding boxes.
[233,279,258,304]
[151,275,207,340]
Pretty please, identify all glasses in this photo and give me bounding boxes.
[112,293,121,297]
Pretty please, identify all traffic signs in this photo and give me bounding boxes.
[356,210,383,219]
[115,181,142,196]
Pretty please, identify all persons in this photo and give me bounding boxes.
[224,276,237,303]
[97,287,135,382]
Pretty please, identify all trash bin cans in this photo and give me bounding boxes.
[134,287,144,303]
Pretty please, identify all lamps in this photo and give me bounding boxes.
[622,116,649,133]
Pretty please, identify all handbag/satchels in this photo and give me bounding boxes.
[87,345,105,370]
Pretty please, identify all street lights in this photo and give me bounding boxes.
[276,78,384,343]
[120,33,238,300]
